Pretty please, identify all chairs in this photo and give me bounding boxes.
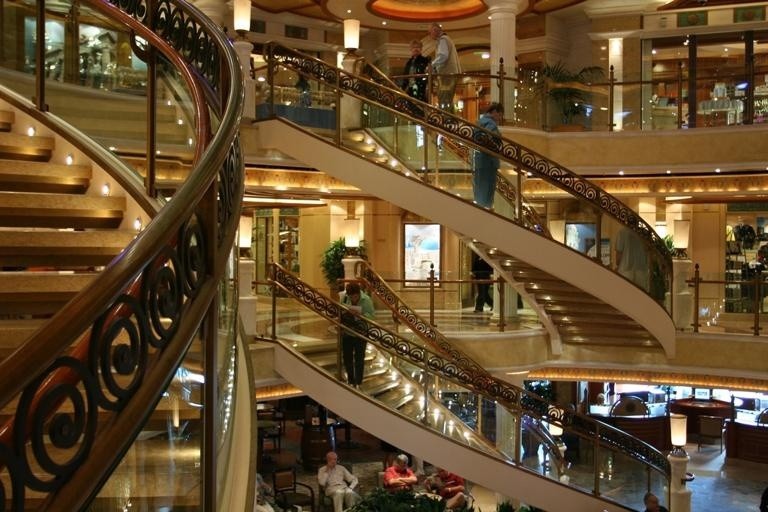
[377,471,414,492]
[697,415,727,453]
[423,473,475,512]
[318,464,352,511]
[260,436,295,469]
[609,396,650,422]
[754,408,768,426]
[271,467,314,511]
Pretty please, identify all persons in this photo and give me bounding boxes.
[470,252,495,314]
[315,451,359,512]
[471,101,502,212]
[255,472,275,512]
[383,455,417,493]
[428,23,462,123]
[594,211,670,307]
[293,65,309,90]
[402,40,431,117]
[641,492,670,511]
[338,281,374,390]
[422,466,464,511]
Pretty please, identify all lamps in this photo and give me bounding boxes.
[670,414,688,457]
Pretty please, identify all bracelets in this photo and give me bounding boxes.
[448,488,450,492]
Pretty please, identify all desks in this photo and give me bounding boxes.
[668,397,737,428]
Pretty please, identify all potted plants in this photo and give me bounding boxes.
[542,62,607,132]
[319,237,348,302]
